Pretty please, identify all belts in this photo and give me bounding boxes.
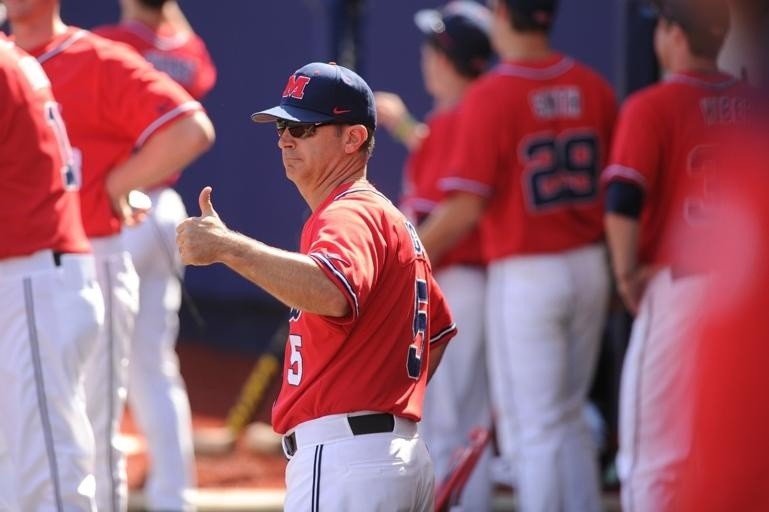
[285,413,394,456]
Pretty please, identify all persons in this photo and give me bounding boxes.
[373,0,494,511]
[0,0,219,511]
[415,0,619,511]
[176,60,457,511]
[599,1,769,512]
[84,1,217,510]
[0,30,104,510]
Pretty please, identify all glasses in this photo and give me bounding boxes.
[274,119,339,140]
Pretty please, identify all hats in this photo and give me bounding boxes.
[657,0,730,38]
[414,1,556,72]
[251,62,376,129]
